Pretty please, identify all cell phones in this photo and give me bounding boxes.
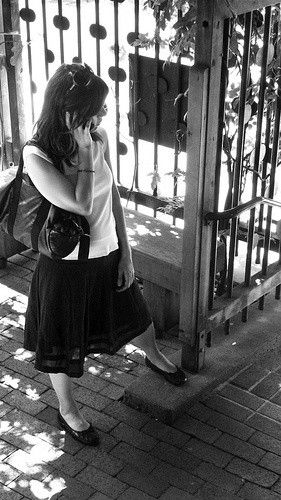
[63,107,96,134]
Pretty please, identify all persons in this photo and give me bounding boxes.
[22,64,189,446]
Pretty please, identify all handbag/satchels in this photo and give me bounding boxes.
[0,138,82,261]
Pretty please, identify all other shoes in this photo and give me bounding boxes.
[145,356,188,385]
[56,408,100,446]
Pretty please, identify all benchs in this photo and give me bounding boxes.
[0,166,226,339]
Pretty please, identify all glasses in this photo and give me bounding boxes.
[65,63,92,96]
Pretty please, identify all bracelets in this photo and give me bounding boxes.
[77,169,95,172]
